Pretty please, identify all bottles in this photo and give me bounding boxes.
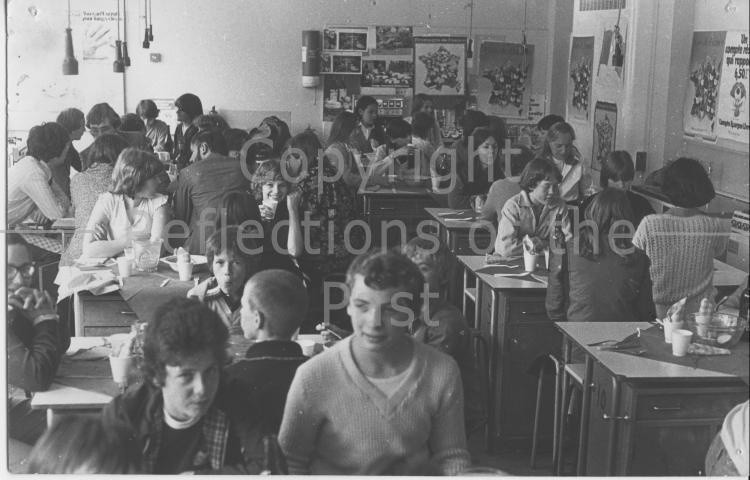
[739,272,750,341]
[163,125,174,159]
[553,215,564,251]
[438,152,452,189]
[209,105,218,116]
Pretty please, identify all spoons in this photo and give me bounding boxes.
[438,212,467,216]
[596,347,650,357]
[446,216,474,221]
[484,264,519,269]
[490,273,529,278]
[587,338,638,347]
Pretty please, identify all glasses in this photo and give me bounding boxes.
[6,264,37,282]
[88,127,110,135]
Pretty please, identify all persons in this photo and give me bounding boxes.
[482,146,537,220]
[540,122,597,201]
[575,150,661,241]
[171,94,204,159]
[108,300,266,475]
[220,268,313,428]
[247,161,307,264]
[6,233,71,445]
[87,104,119,145]
[121,114,152,150]
[71,133,135,255]
[8,122,74,264]
[406,113,438,187]
[636,158,732,327]
[547,187,659,335]
[457,95,494,143]
[84,146,167,279]
[368,120,434,188]
[288,134,355,261]
[179,129,261,238]
[449,125,511,213]
[322,110,365,193]
[187,229,259,329]
[351,96,384,164]
[193,115,253,234]
[280,250,472,476]
[134,99,171,161]
[495,155,575,260]
[402,94,445,128]
[536,114,565,150]
[51,108,87,183]
[28,413,134,475]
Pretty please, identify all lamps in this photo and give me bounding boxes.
[143,0,149,49]
[612,0,623,67]
[122,0,130,66]
[522,0,528,53]
[467,0,474,58]
[148,0,154,40]
[62,0,79,75]
[113,0,124,73]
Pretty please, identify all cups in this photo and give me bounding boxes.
[169,164,177,174]
[132,239,162,273]
[524,254,538,272]
[178,261,194,281]
[117,255,133,279]
[263,199,278,220]
[671,328,693,357]
[406,142,417,157]
[300,340,315,357]
[663,320,684,343]
[110,354,132,384]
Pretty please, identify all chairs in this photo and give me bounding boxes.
[531,321,597,473]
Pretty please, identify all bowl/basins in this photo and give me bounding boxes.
[321,340,342,350]
[228,325,252,359]
[687,311,746,348]
[160,255,209,273]
